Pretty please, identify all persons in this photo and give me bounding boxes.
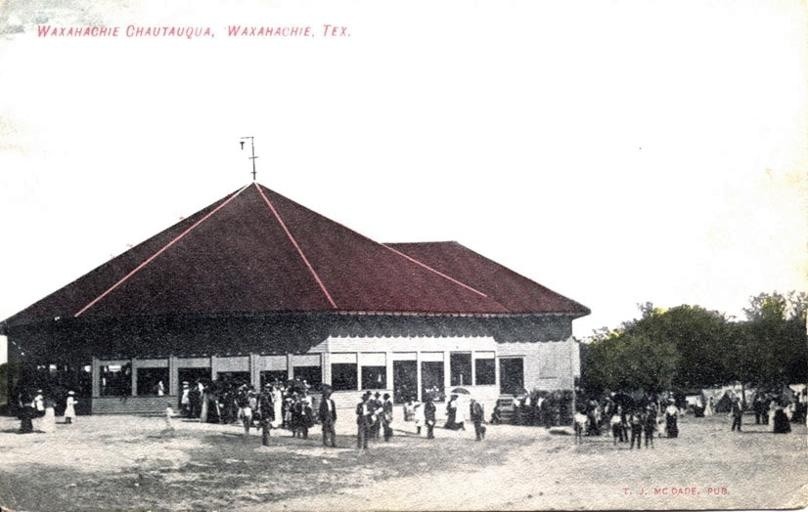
[16,365,801,448]
